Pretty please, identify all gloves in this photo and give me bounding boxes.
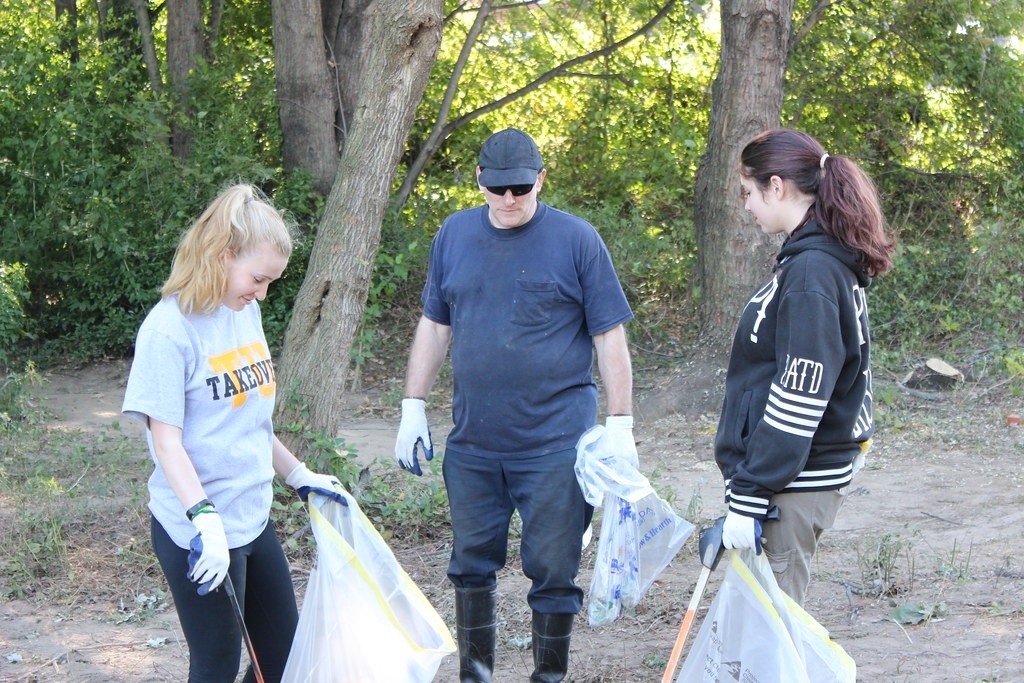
[605,415,639,476]
[284,459,347,506]
[186,503,231,596]
[722,510,763,556]
[394,396,433,476]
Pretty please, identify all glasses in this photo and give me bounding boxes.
[486,186,533,196]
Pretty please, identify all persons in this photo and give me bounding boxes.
[714,129,897,611]
[121,183,348,683]
[394,128,640,683]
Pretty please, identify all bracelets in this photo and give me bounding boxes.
[186,498,218,521]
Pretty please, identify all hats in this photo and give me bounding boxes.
[478,128,541,187]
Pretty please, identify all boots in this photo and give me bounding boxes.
[529,604,575,683]
[454,583,498,682]
